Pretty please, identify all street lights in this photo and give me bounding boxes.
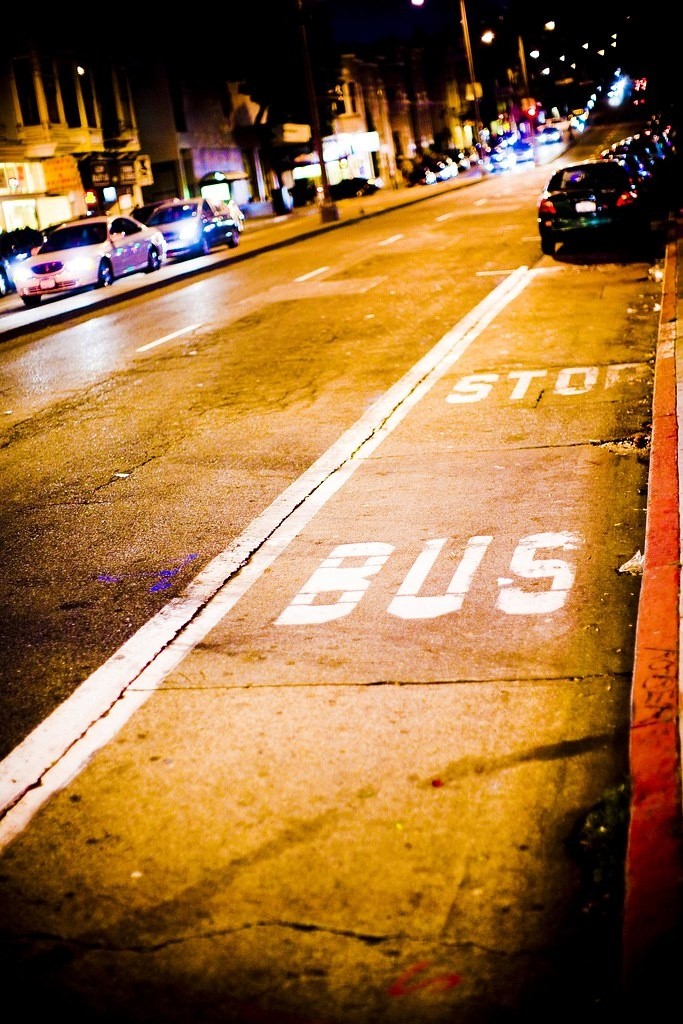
[480,19,555,166]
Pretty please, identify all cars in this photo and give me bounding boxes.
[409,108,683,256]
[140,196,240,263]
[329,177,384,202]
[8,214,168,308]
